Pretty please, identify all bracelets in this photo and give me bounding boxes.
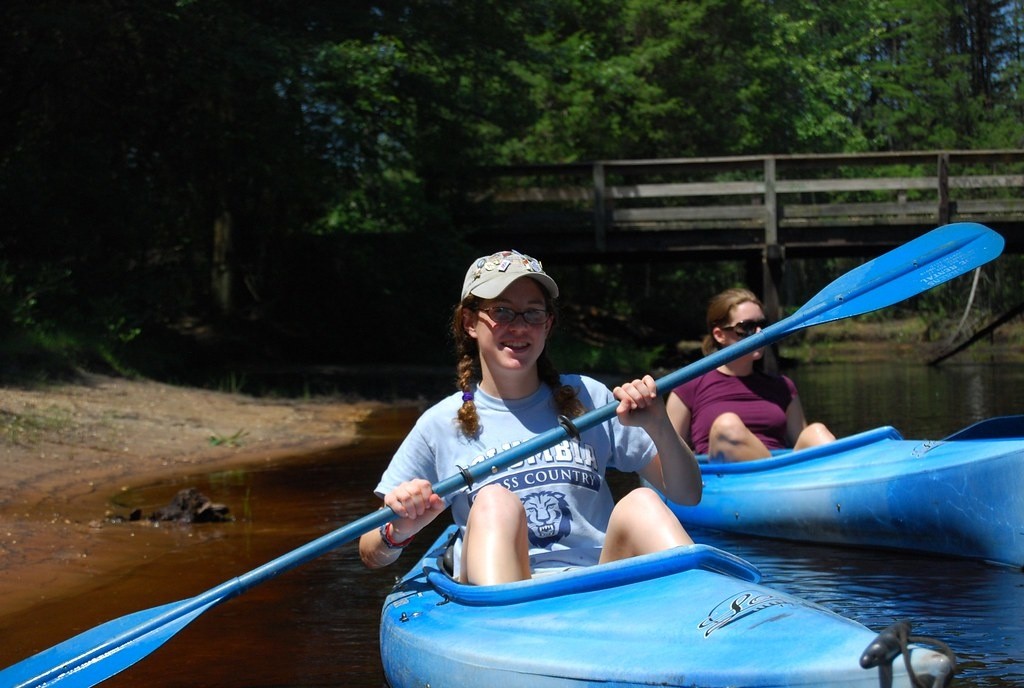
[378,522,415,549]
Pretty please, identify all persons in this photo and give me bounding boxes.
[359,250,702,585]
[666,288,836,463]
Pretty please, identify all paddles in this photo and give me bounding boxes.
[943,412,1024,442]
[0,221,1006,688]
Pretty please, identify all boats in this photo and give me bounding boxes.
[375,521,956,688]
[639,414,1024,569]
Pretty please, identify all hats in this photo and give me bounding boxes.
[461,250,558,303]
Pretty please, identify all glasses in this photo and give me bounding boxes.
[477,306,552,325]
[720,319,768,337]
